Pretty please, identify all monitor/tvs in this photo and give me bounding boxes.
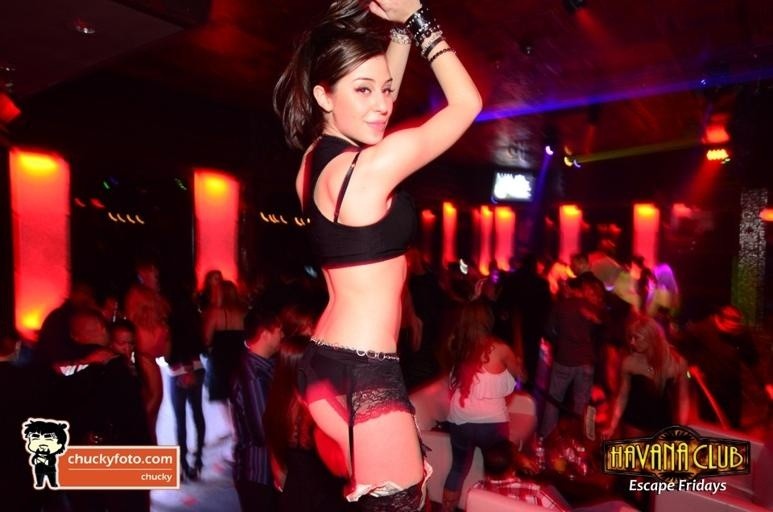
[489,169,538,204]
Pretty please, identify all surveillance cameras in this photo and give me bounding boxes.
[520,42,537,56]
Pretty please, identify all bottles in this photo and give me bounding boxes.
[535,442,546,471]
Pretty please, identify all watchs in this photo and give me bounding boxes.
[272,0,482,512]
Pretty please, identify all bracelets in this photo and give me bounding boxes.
[389,7,456,63]
